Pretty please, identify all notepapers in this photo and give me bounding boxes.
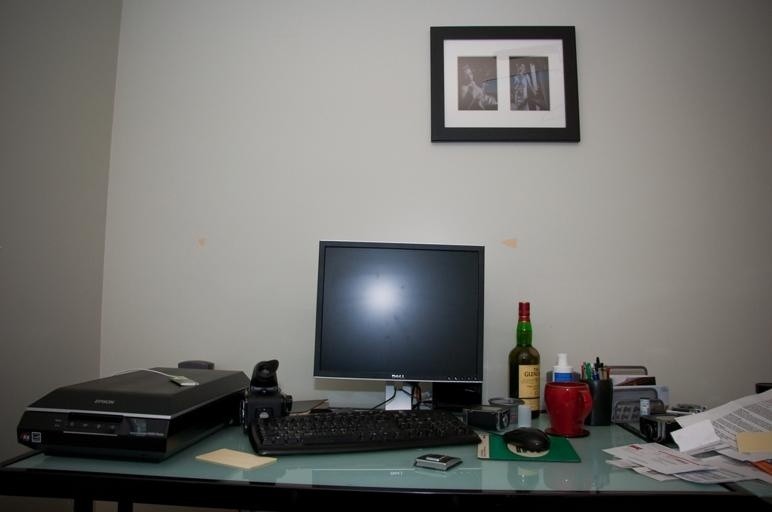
[736,431,772,455]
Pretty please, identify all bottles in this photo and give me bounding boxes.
[507,302,540,418]
[552,352,575,384]
[638,396,650,417]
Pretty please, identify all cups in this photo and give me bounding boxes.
[489,396,524,435]
[545,382,592,435]
[585,378,614,427]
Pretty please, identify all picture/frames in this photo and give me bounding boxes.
[429,27,582,143]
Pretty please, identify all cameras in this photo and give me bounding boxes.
[241,360,292,434]
[640,413,691,445]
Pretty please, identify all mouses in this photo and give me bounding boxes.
[503,427,550,457]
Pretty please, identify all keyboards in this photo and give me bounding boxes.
[248,410,482,456]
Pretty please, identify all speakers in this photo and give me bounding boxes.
[432,382,482,414]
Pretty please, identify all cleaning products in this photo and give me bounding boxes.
[554,353,572,382]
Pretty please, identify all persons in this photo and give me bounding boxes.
[513,62,537,110]
[460,64,497,110]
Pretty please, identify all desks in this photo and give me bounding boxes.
[0,408,732,512]
[622,421,772,512]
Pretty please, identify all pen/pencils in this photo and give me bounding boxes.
[581,357,611,382]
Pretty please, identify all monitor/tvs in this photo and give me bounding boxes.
[313,241,485,411]
